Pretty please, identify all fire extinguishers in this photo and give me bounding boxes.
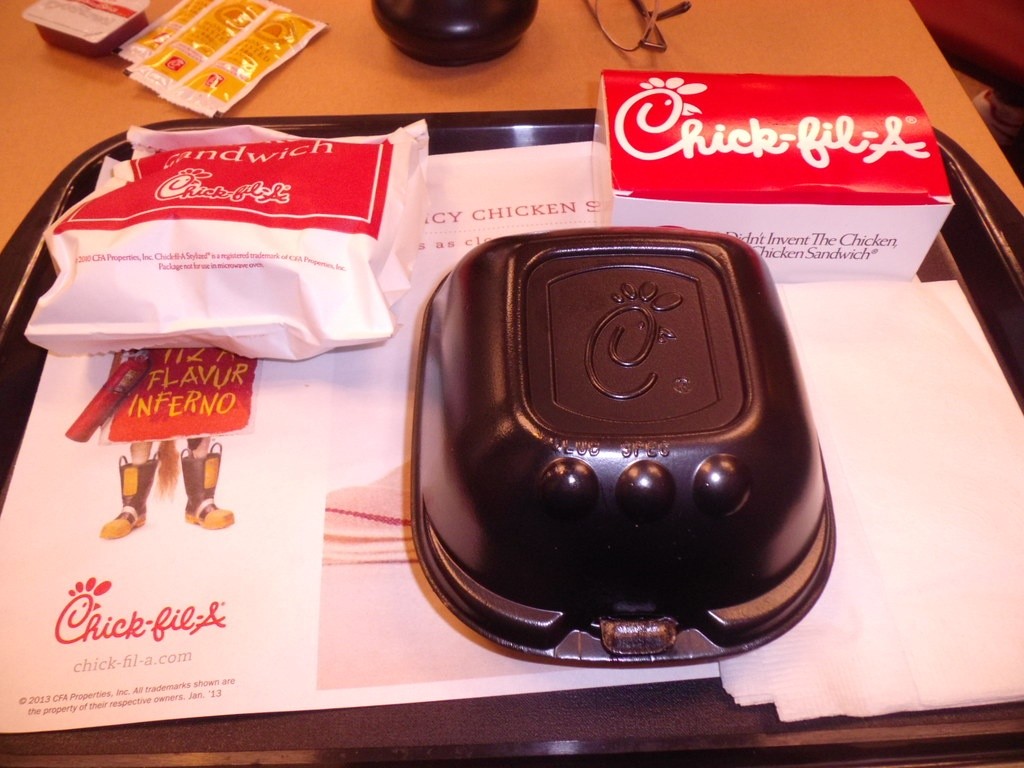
[65,349,150,442]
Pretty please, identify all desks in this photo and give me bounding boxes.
[1,0,1024,253]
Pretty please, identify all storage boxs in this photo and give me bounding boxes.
[591,69,955,285]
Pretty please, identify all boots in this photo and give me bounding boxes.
[180,442,234,530]
[99,453,161,537]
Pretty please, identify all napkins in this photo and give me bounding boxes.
[719,278,1023,725]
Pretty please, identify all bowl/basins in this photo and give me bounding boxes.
[371,0,538,68]
[411,226,836,669]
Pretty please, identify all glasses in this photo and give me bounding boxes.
[583,0,690,52]
